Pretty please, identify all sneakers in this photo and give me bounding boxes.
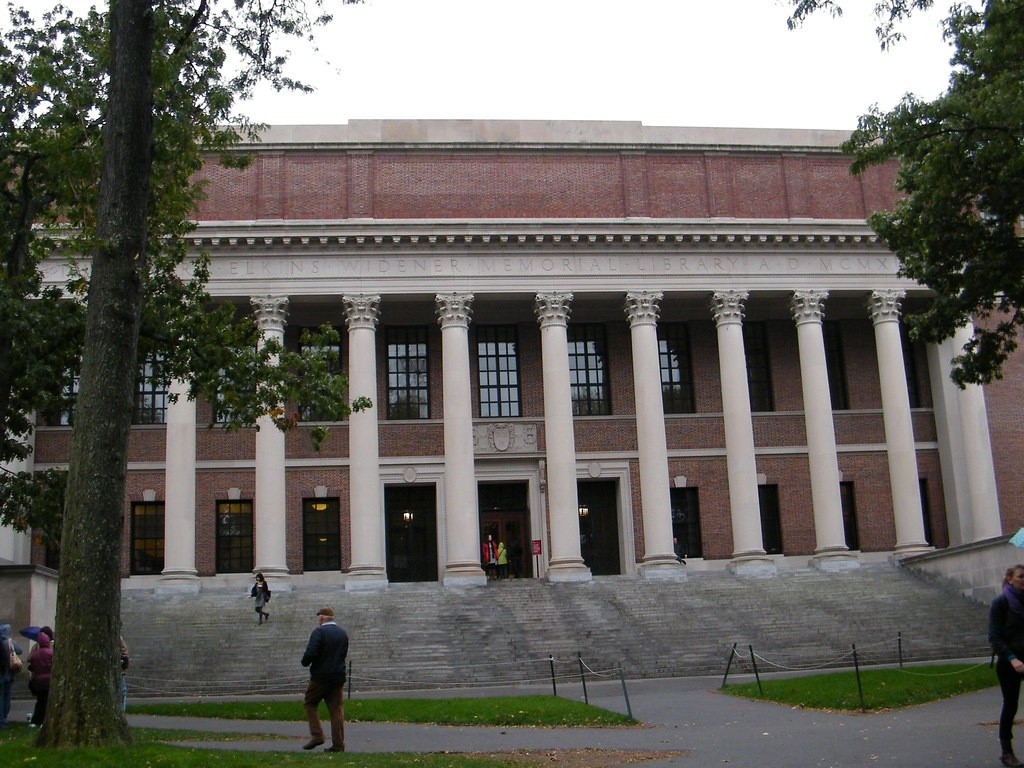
[1002,752,1024,768]
[324,745,345,752]
[302,739,323,750]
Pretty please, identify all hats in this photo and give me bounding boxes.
[316,608,334,616]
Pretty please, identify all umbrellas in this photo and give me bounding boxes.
[18,626,42,649]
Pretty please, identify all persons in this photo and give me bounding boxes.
[0,623,23,728]
[119,621,129,725]
[481,533,524,579]
[674,538,686,565]
[301,607,348,752]
[989,564,1024,768]
[26,626,54,727]
[251,573,271,625]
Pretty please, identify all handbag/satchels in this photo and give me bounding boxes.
[28,677,39,694]
[8,638,23,671]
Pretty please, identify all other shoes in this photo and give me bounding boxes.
[265,613,269,621]
[258,621,263,625]
[488,575,498,580]
[0,719,10,725]
[30,723,43,729]
